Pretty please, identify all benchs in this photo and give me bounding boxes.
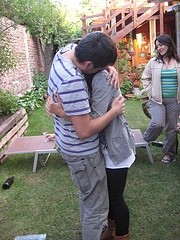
[5,129,154,172]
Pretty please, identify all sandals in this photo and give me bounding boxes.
[161,155,171,163]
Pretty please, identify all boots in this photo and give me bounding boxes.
[100,218,116,240]
[110,228,131,240]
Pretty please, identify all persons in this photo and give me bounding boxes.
[45,66,137,240]
[48,31,125,240]
[142,35,180,163]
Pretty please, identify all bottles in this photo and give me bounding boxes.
[2,176,14,189]
